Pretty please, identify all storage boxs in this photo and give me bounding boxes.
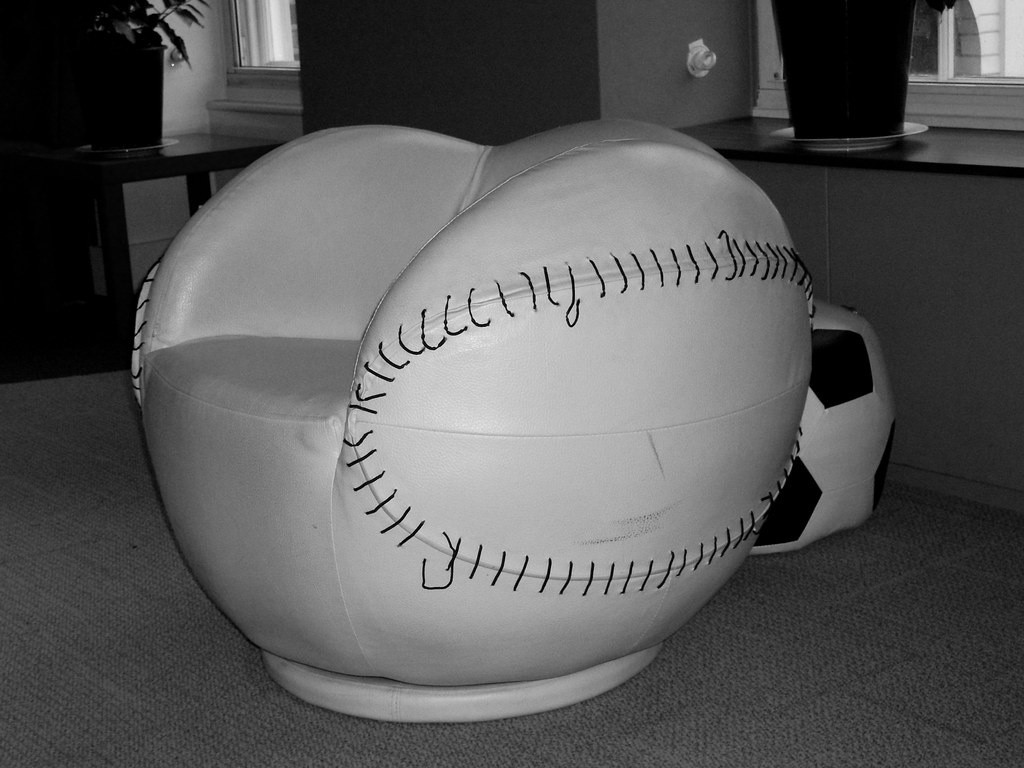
[296,0,760,148]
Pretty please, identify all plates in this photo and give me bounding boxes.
[767,120,930,151]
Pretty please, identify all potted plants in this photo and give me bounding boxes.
[65,0,200,148]
[768,0,958,138]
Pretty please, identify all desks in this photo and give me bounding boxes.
[0,141,285,370]
